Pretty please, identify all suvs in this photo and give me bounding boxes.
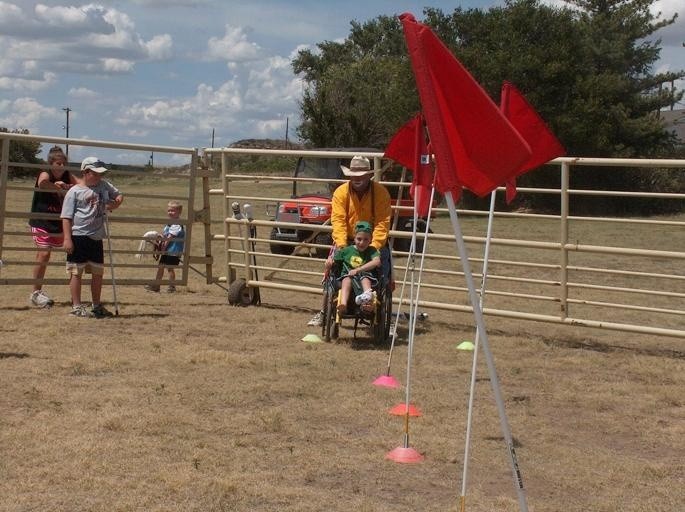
[266,149,436,256]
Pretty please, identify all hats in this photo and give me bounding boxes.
[81,157,108,174]
[355,221,372,233]
[340,156,381,176]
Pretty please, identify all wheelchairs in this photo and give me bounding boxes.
[322,253,395,341]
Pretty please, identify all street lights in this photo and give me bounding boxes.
[60,106,72,160]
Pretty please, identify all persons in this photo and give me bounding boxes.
[146,201,187,294]
[61,157,122,319]
[28,145,96,308]
[326,224,376,316]
[309,159,396,332]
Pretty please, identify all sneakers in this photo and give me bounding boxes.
[69,305,87,317]
[307,313,323,325]
[30,290,53,306]
[91,305,113,316]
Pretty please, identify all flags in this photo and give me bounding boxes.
[380,112,459,219]
[399,10,516,198]
[492,79,565,206]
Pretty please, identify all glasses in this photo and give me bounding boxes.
[83,161,105,168]
